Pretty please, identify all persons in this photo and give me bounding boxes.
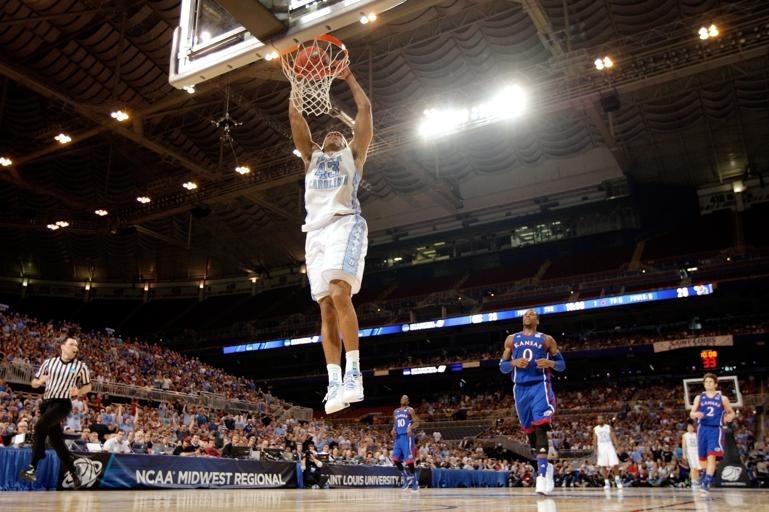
[0,302,769,495]
[287,61,374,416]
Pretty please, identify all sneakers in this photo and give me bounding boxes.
[614,479,623,488]
[545,463,555,494]
[411,474,420,490]
[603,483,610,489]
[321,382,350,415]
[341,370,366,404]
[21,464,38,482]
[71,463,83,489]
[535,475,546,495]
[401,475,412,491]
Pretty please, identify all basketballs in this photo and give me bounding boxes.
[292,45,330,81]
